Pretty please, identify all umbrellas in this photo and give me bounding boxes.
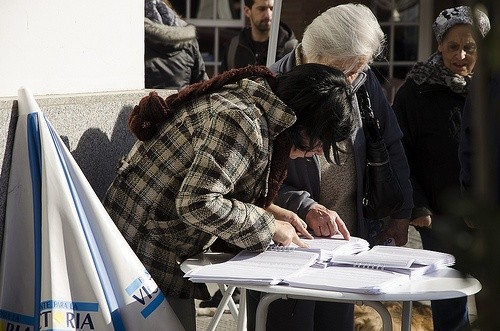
[0,89,187,331]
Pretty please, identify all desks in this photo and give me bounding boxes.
[178,256,482,331]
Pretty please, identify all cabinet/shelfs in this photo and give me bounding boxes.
[182,0,246,76]
[371,22,420,90]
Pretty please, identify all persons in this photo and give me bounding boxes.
[223,0,298,71]
[247,4,414,331]
[102,64,357,331]
[392,7,500,331]
[145,0,209,89]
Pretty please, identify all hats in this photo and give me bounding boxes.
[431,5,491,46]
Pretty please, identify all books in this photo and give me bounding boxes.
[183,231,456,294]
